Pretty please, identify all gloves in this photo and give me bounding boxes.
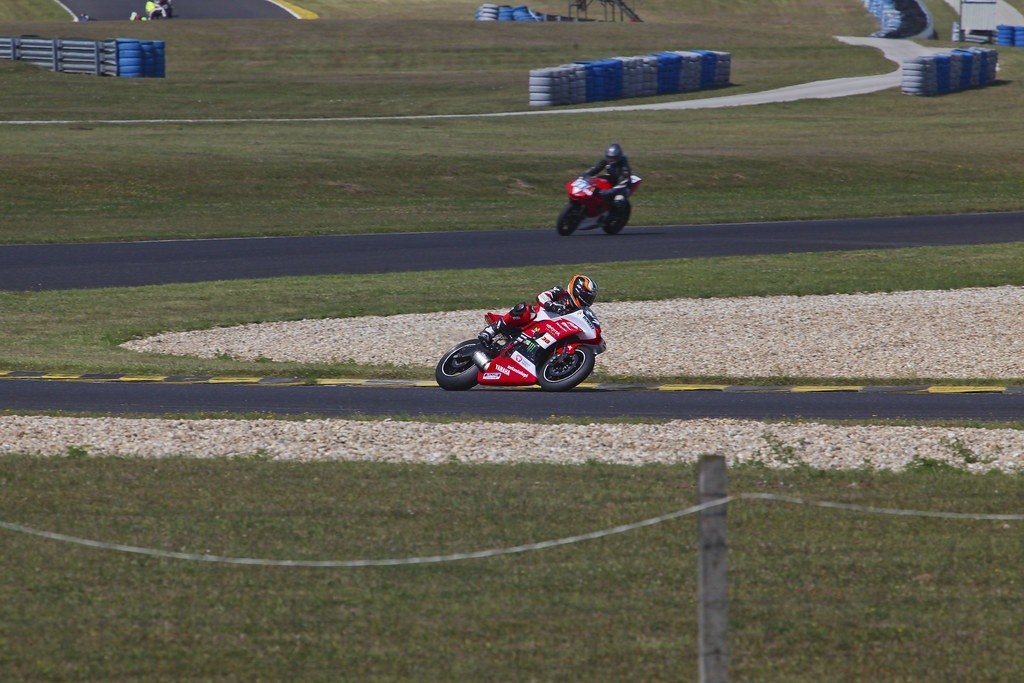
[598,189,614,197]
[545,300,566,316]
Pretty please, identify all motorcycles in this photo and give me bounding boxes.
[145,0,174,19]
[434,303,607,393]
[556,171,643,236]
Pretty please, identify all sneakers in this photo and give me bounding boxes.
[478,327,493,350]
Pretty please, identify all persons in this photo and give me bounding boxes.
[478,274,597,345]
[579,143,630,220]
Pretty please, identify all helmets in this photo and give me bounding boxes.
[567,274,598,309]
[605,143,623,164]
